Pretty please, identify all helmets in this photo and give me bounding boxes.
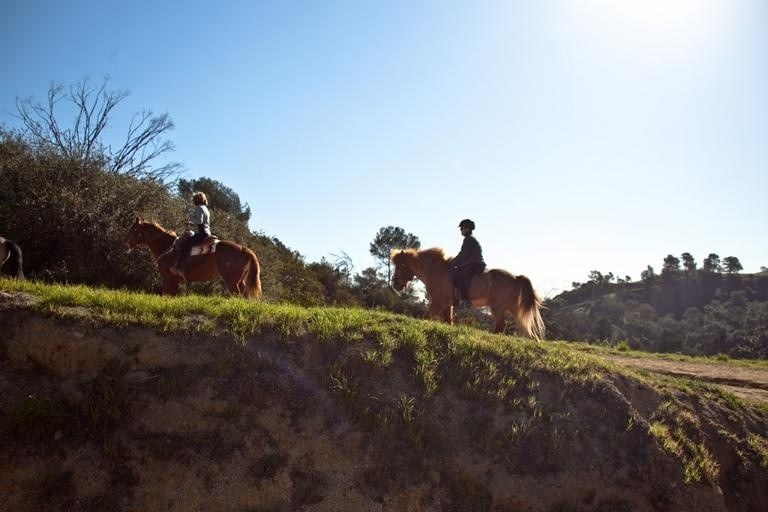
[458,219,475,229]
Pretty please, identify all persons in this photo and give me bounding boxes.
[446,218,486,310]
[174,190,210,272]
[180,218,195,242]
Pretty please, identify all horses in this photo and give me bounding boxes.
[122,215,262,301]
[389,248,549,343]
[0,236,24,280]
